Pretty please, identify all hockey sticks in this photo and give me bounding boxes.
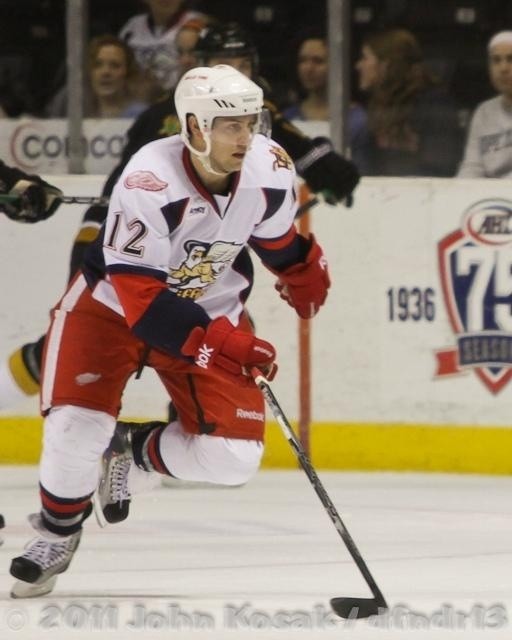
[251,366,388,620]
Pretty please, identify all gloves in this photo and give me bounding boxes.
[271,230,335,322]
[289,134,365,212]
[0,159,65,225]
[178,313,280,393]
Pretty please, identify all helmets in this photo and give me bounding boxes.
[171,64,264,138]
[185,17,262,80]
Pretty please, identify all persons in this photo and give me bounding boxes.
[1,0,511,224]
[9,63,333,585]
[10,24,359,423]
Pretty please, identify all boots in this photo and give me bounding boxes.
[90,416,165,530]
[7,509,87,600]
